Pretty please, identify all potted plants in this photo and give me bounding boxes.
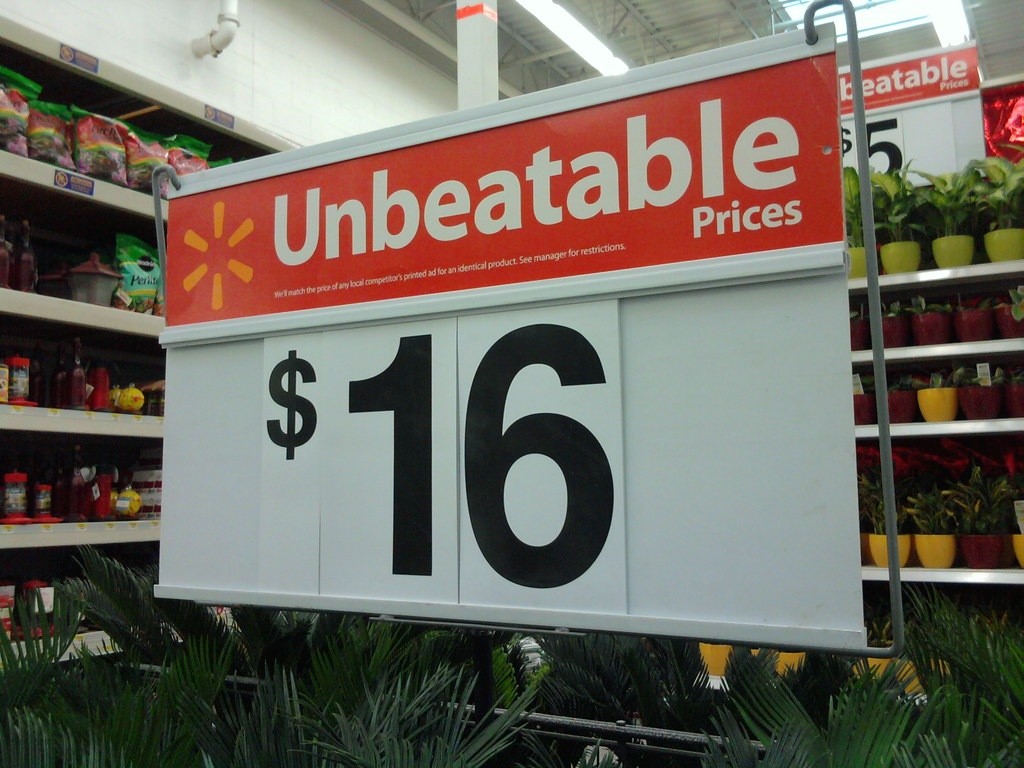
[869,159,927,275]
[912,366,966,421]
[850,303,869,351]
[957,363,1004,420]
[901,161,981,269]
[905,295,952,345]
[888,375,917,423]
[996,286,1024,338]
[1005,367,1024,418]
[906,482,958,568]
[853,377,878,425]
[857,474,910,568]
[1010,491,1024,569]
[881,301,908,347]
[955,290,993,342]
[843,166,867,280]
[974,157,1024,264]
[954,466,1012,569]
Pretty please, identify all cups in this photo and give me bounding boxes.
[88,362,111,412]
[0,364,9,402]
[95,468,111,518]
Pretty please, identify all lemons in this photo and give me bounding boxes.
[109,491,143,515]
[109,386,144,411]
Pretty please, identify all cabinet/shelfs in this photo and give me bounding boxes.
[0,14,306,672]
[693,259,1024,705]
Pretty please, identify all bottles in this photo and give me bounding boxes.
[50,340,69,408]
[16,220,37,292]
[70,336,85,408]
[0,215,10,288]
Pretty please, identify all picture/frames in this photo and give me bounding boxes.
[0,574,54,638]
